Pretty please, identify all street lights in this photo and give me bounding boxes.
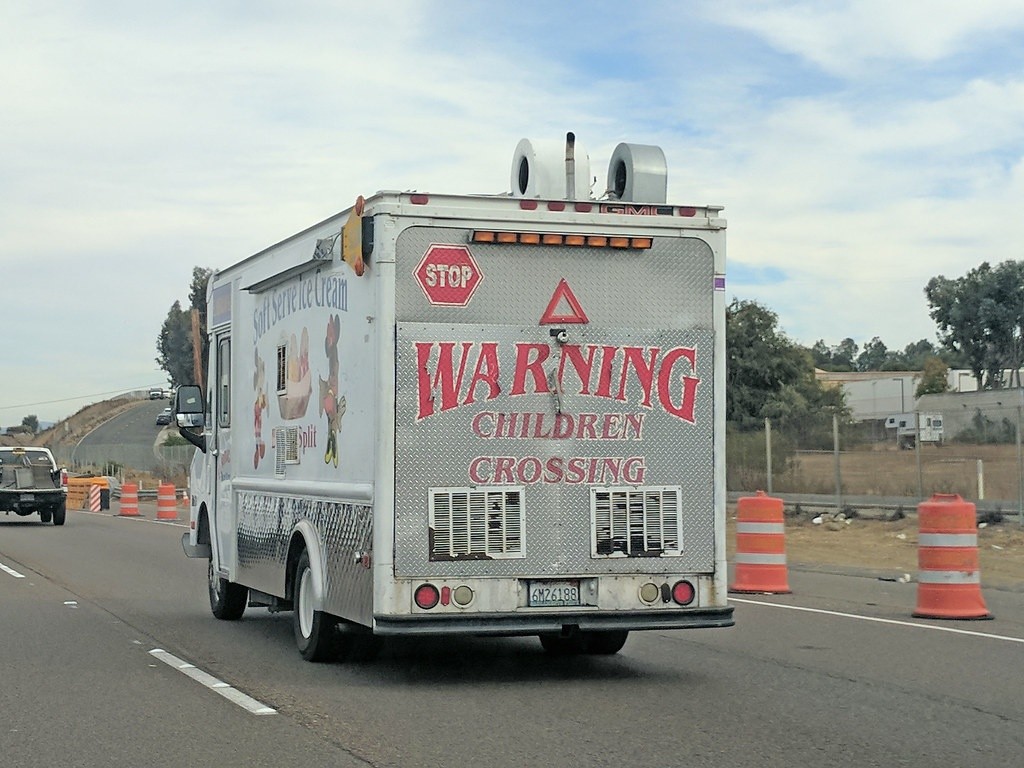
[892,377,904,414]
[958,372,970,393]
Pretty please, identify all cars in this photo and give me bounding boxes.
[156,414,171,425]
[163,407,171,415]
[162,389,176,400]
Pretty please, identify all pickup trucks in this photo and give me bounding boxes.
[0,445,69,526]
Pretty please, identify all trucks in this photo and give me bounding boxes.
[149,388,163,400]
[175,129,736,662]
